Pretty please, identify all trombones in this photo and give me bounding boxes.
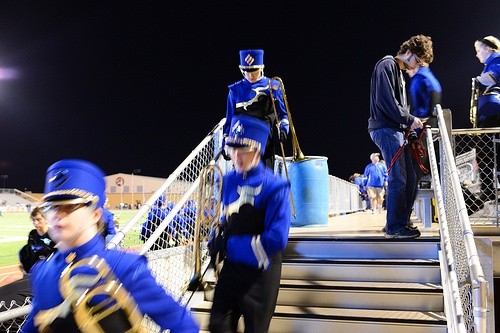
[268,77,309,220]
[59,254,148,333]
[469,77,479,128]
[187,165,220,302]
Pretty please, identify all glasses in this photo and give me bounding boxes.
[414,57,426,68]
[45,205,80,212]
[225,145,255,154]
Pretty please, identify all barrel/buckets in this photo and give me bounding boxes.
[280,155,329,227]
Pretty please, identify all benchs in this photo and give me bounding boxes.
[416,189,433,228]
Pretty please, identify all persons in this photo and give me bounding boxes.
[139,193,217,250]
[205,116,294,333]
[366,34,437,239]
[222,47,290,176]
[381,61,442,232]
[472,35,500,203]
[19,206,58,278]
[348,152,388,215]
[18,160,199,333]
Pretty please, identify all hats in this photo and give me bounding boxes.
[370,152,380,160]
[225,116,270,155]
[238,49,265,71]
[39,159,107,210]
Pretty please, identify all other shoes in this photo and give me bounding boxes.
[371,208,382,214]
[382,221,422,239]
